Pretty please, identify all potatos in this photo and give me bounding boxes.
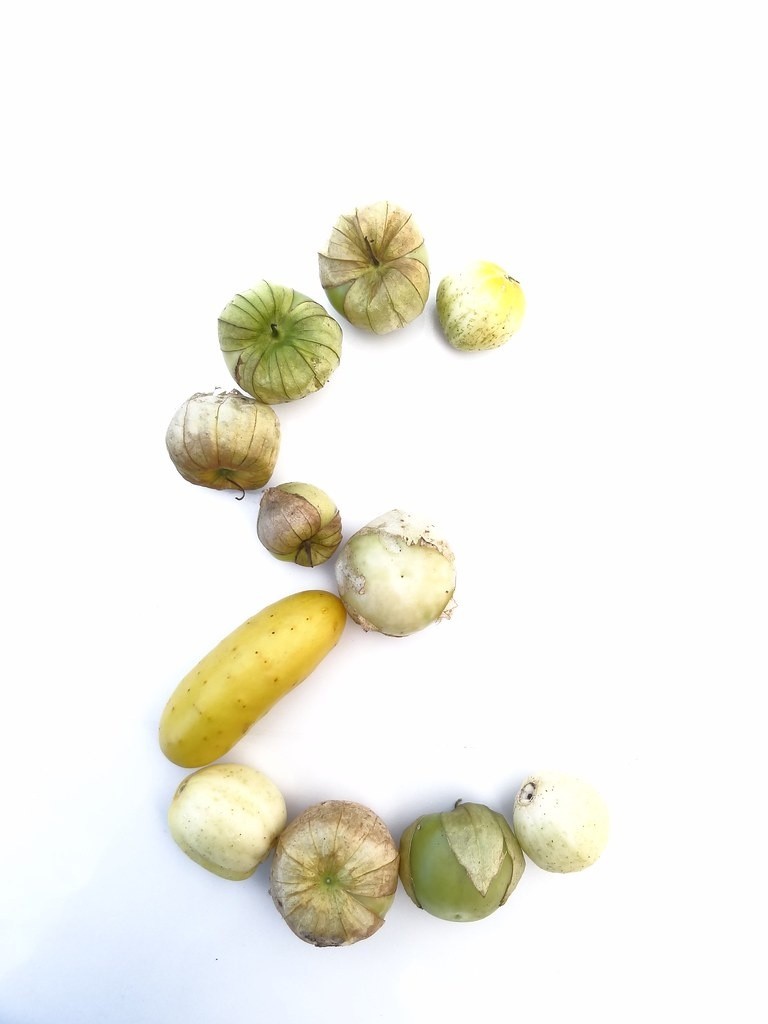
[159,591,346,768]
